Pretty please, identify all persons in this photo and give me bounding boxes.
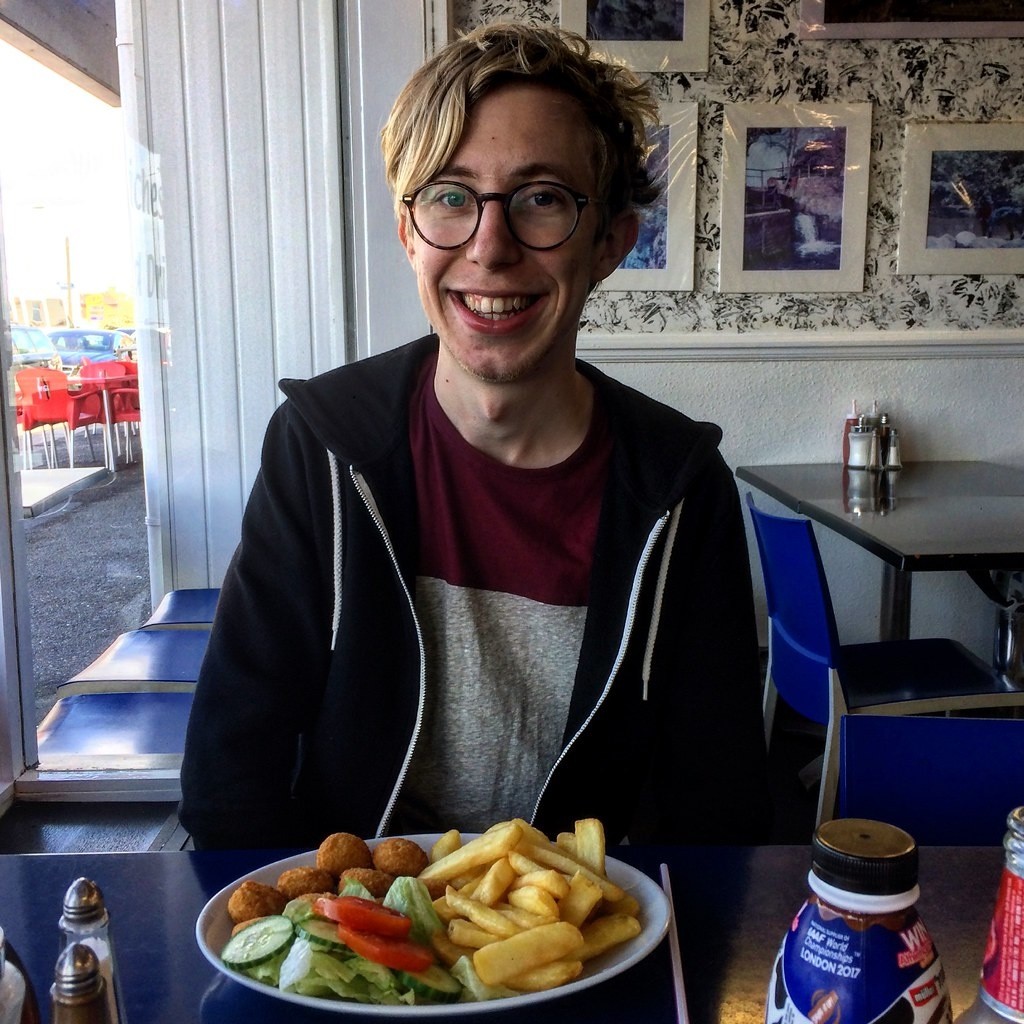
[174,24,767,850]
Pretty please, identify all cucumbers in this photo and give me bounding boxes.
[220,915,296,969]
[293,918,348,948]
[391,966,462,1003]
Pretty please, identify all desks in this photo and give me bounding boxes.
[21,467,108,519]
[40,374,138,473]
[735,460,1024,643]
[0,844,1002,1024]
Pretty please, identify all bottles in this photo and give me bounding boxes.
[952,806,1024,1024]
[763,817,953,1024]
[843,398,902,472]
[55,877,116,986]
[0,925,30,1024]
[49,941,115,1023]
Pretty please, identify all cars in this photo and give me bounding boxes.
[49,328,137,371]
[2,326,63,376]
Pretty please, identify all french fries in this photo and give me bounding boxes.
[414,817,640,996]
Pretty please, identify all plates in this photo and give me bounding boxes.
[194,833,672,1017]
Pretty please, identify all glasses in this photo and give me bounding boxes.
[397,176,607,251]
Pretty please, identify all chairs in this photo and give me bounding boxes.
[15,360,141,473]
[839,714,1024,847]
[744,491,1024,831]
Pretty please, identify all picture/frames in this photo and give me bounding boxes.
[599,101,699,291]
[799,0,1024,40]
[896,122,1024,276]
[718,102,873,293]
[559,0,710,73]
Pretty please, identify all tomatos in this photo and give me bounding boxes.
[337,923,433,973]
[311,894,411,938]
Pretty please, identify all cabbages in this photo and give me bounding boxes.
[239,873,518,1005]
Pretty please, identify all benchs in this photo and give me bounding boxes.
[15,588,222,804]
